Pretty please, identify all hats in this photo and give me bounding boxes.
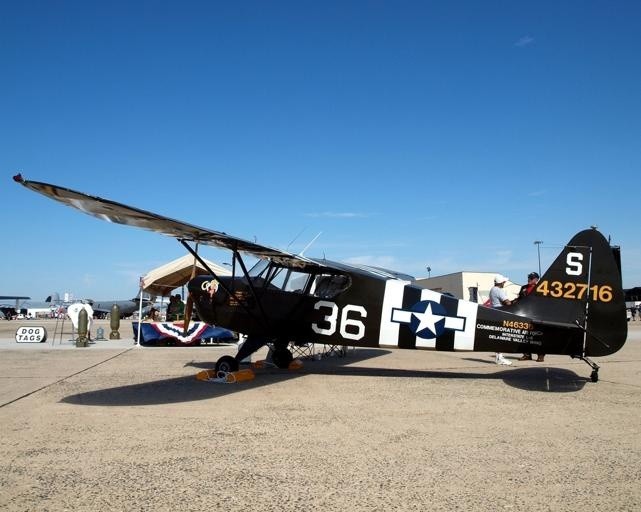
[494,274,509,284]
[528,272,540,279]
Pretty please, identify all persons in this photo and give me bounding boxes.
[490,275,512,366]
[519,272,544,362]
[166,294,184,321]
[631,305,641,321]
[7,311,11,321]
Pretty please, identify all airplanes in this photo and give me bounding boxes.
[13,173,628,384]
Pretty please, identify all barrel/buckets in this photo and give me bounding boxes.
[96,326,103,339]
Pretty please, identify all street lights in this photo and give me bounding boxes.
[425,263,433,279]
[534,239,544,281]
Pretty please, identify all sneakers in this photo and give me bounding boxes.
[495,354,512,365]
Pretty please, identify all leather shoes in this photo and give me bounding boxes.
[519,355,533,361]
[536,353,545,362]
